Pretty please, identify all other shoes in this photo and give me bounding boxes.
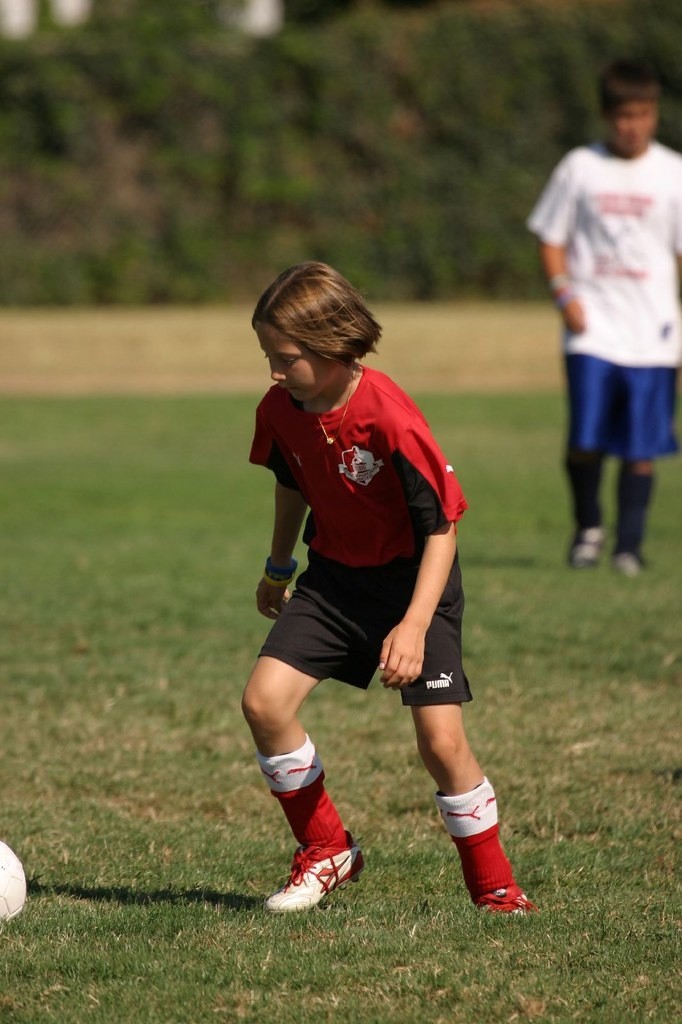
[612,550,641,578]
[567,528,603,571]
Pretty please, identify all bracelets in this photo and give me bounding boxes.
[547,274,567,291]
[557,289,576,308]
[264,558,297,587]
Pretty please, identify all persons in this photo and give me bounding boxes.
[241,260,535,920]
[524,59,682,577]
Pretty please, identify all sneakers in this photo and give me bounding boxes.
[266,830,364,913]
[477,887,539,918]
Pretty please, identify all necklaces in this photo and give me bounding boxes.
[311,365,355,444]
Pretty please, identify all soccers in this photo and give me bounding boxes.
[0,842,27,920]
